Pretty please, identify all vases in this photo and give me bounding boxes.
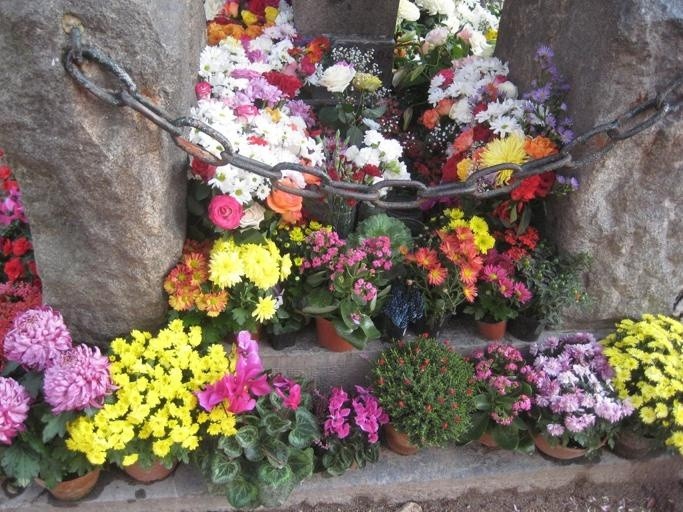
[312,311,359,352]
[477,313,547,339]
